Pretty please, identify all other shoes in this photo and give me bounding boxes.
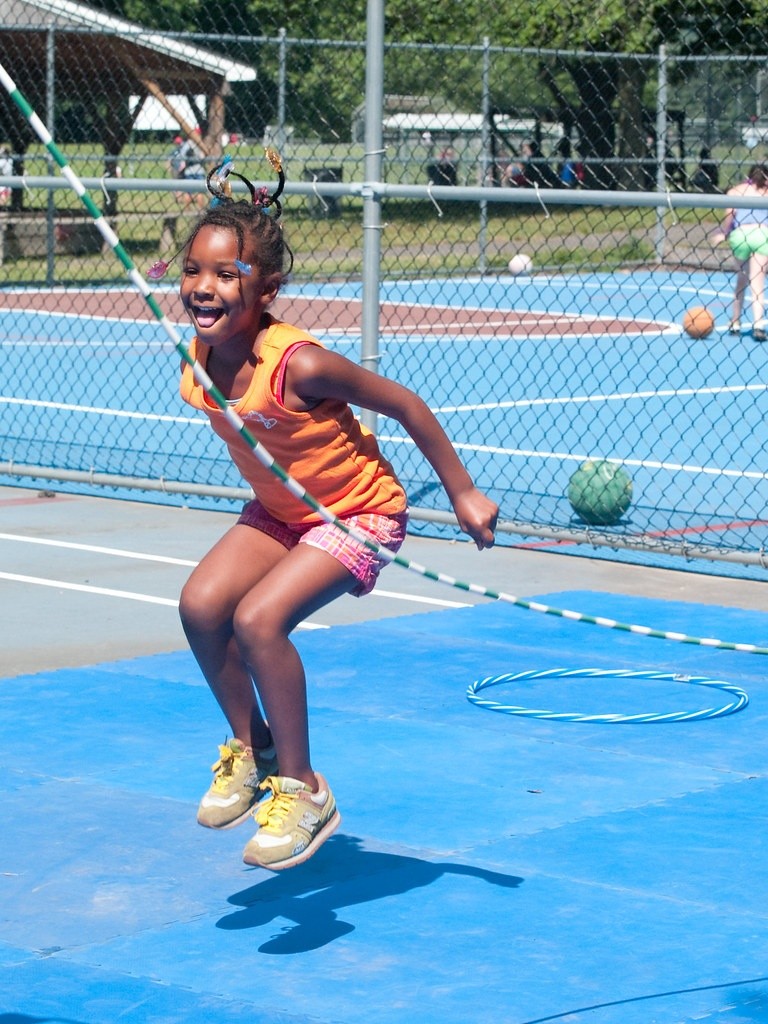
[754,327,767,341]
[728,322,741,337]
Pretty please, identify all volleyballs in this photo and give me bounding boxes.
[566,460,634,526]
[507,253,534,277]
[683,306,716,339]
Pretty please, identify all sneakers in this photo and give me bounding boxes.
[242,769,341,872]
[197,722,280,831]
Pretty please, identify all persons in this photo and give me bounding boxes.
[164,122,229,212]
[479,138,718,193]
[426,144,456,185]
[0,147,29,205]
[423,129,434,154]
[713,164,768,341]
[178,148,499,870]
